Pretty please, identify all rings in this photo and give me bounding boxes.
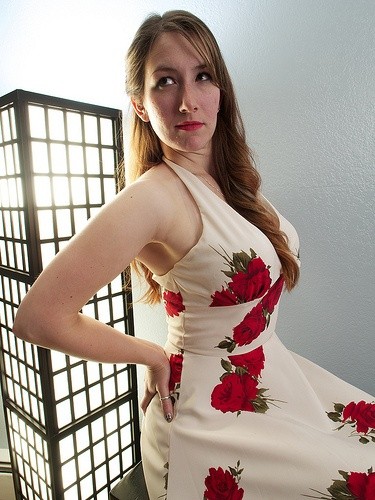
[160,396,170,400]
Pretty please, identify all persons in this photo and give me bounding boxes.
[12,10,375,500]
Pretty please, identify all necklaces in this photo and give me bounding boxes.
[199,174,223,195]
